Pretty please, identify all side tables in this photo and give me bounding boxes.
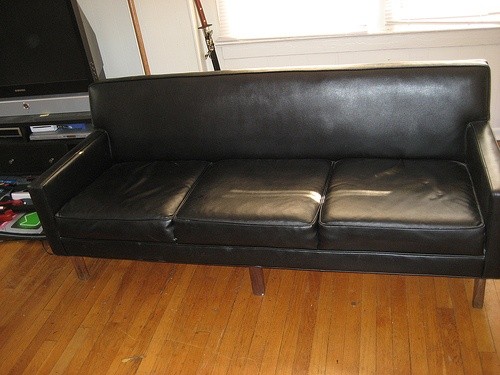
[0,111,94,242]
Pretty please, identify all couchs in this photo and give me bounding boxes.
[28,58,500,310]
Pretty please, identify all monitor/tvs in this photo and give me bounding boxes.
[0,0,106,117]
